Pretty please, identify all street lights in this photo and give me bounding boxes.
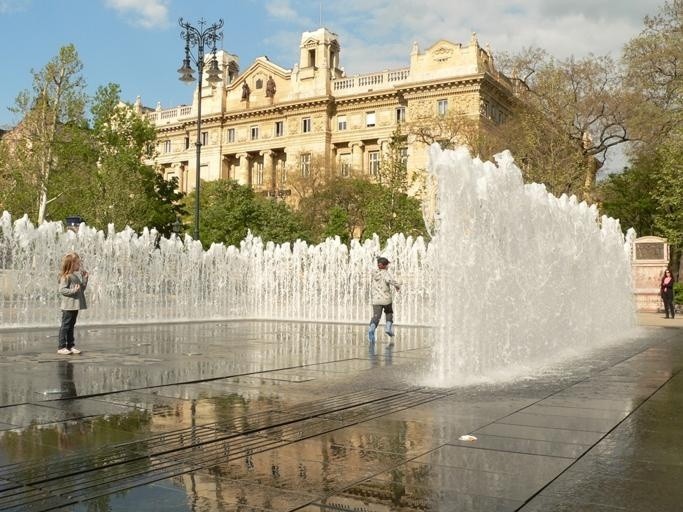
[175,16,226,245]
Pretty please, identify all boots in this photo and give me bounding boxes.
[368,324,376,341]
[385,321,395,337]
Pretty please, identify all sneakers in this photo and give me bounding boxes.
[69,347,80,354]
[56,348,73,355]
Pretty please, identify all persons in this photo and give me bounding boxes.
[659,269,675,318]
[367,258,401,341]
[57,253,89,354]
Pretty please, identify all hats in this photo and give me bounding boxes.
[377,257,390,267]
